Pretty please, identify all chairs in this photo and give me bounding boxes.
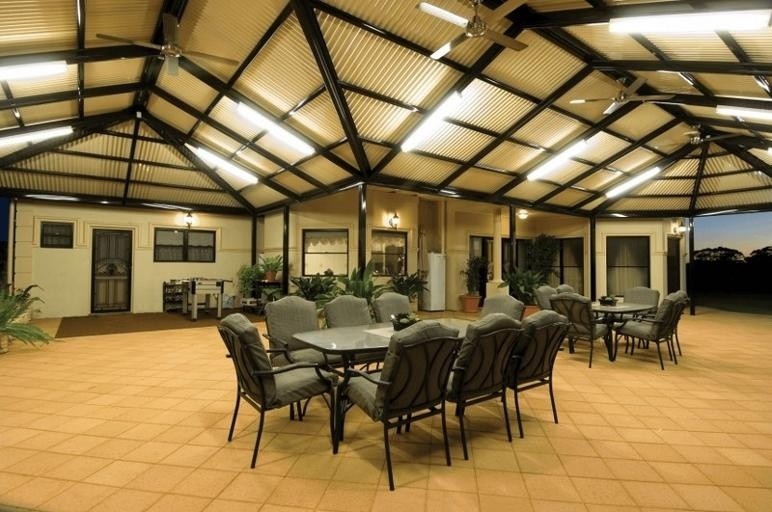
[549,292,609,368]
[505,310,572,439]
[532,286,557,309]
[638,290,690,361]
[262,296,343,421]
[556,284,574,294]
[614,287,660,340]
[405,313,522,460]
[325,295,373,371]
[479,294,527,321]
[340,320,459,490]
[372,292,411,369]
[613,293,684,370]
[217,313,338,468]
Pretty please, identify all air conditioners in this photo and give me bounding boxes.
[422,251,445,311]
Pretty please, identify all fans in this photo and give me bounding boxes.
[415,0,529,60]
[570,75,675,115]
[654,124,743,145]
[96,13,240,77]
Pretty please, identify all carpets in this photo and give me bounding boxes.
[54,308,266,338]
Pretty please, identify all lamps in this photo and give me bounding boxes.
[0,53,68,87]
[185,212,192,230]
[235,102,316,158]
[527,139,587,184]
[605,167,660,198]
[401,91,463,153]
[0,126,73,152]
[609,9,771,35]
[195,148,259,186]
[517,209,529,220]
[717,105,772,121]
[389,210,401,230]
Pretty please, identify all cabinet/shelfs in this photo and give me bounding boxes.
[162,281,189,313]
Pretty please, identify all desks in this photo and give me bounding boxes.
[592,303,656,361]
[292,319,476,455]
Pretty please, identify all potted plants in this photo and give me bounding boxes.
[497,265,545,320]
[0,283,65,354]
[459,256,482,313]
[237,264,265,298]
[255,254,283,282]
